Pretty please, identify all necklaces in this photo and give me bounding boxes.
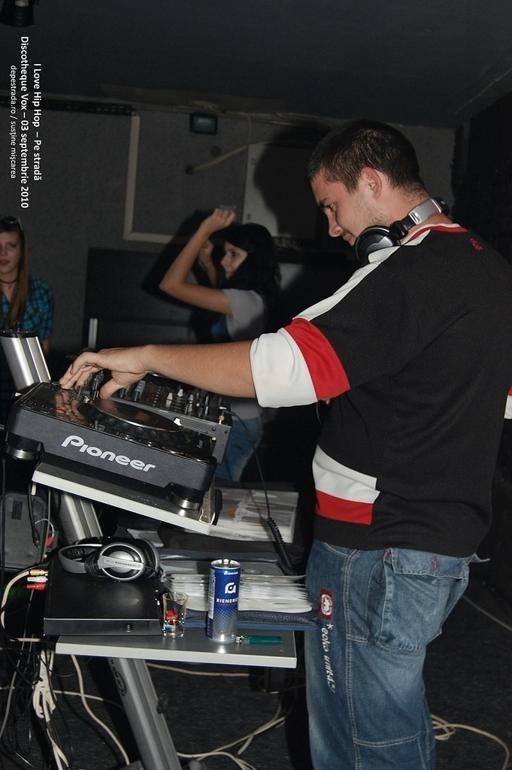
[0,276,19,285]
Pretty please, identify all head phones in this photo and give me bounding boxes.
[58,537,160,582]
[355,196,447,266]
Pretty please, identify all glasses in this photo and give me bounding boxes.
[4,214,23,232]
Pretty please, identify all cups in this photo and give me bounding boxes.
[162,592,189,638]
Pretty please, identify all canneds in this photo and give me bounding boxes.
[204,557,242,644]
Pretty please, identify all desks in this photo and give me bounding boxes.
[56,483,300,770]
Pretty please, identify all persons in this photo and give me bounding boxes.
[0,215,55,360]
[60,116,512,770]
[157,205,282,488]
